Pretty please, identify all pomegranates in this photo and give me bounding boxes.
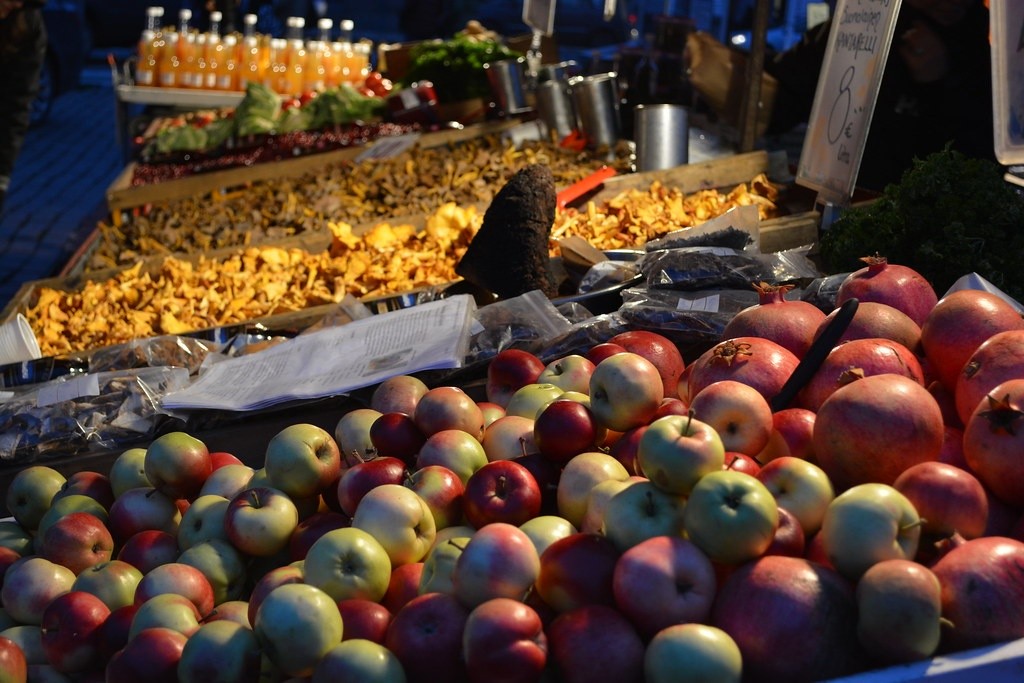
[608,251,1024,647]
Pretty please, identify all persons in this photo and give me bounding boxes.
[857,0,1004,185]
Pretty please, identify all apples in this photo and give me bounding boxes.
[0,339,955,683]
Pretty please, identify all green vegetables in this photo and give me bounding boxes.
[152,79,387,151]
[392,38,519,104]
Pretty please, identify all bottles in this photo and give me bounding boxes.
[137,8,368,99]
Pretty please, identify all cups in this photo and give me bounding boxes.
[539,79,580,141]
[486,61,523,113]
[637,104,690,170]
[570,73,616,148]
